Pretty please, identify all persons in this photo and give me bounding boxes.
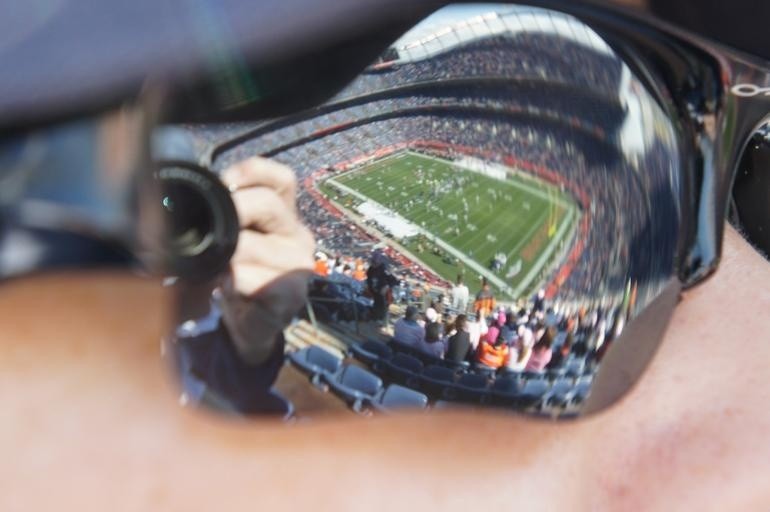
[0,0,770,511]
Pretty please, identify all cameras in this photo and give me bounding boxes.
[101,156,239,328]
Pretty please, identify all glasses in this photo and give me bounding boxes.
[0,0,770,297]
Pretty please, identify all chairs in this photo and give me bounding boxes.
[280,263,618,421]
